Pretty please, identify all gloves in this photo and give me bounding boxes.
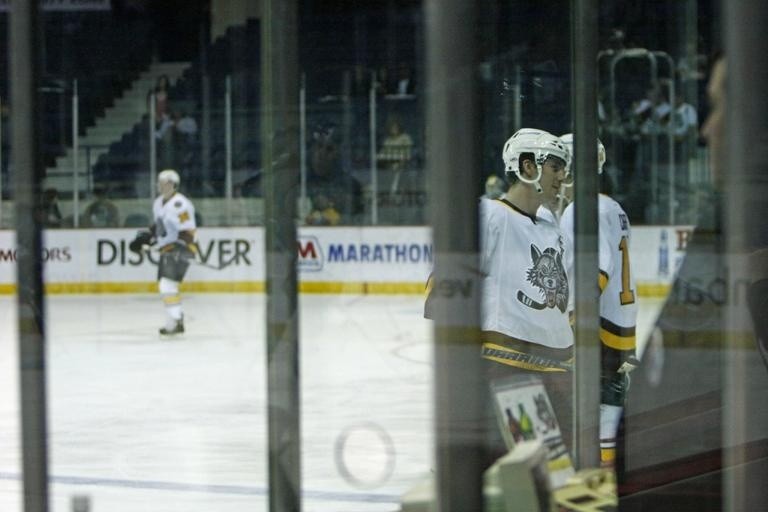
[130,232,157,252]
[174,230,194,265]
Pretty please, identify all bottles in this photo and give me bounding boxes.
[659,230,669,276]
[505,402,539,445]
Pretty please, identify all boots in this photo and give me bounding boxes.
[160,312,184,334]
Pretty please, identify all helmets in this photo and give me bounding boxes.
[176,117,199,136]
[502,126,607,186]
[158,168,181,184]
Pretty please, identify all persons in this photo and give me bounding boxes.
[177,94,202,147]
[589,26,714,183]
[146,73,172,121]
[553,117,638,469]
[424,119,573,456]
[608,40,768,512]
[126,167,198,338]
[376,111,414,197]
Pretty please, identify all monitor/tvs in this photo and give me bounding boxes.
[481,439,555,512]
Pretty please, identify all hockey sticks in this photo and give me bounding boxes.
[142,245,236,270]
[482,347,640,380]
